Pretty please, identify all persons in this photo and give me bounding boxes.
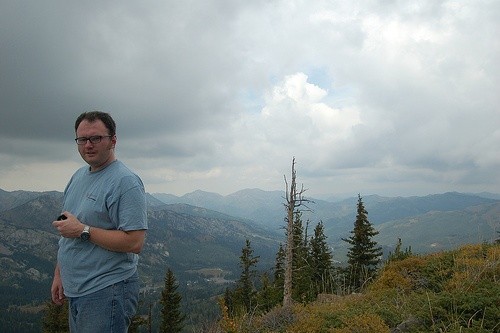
[50,108,149,333]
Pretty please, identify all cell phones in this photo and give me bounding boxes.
[57,214,67,221]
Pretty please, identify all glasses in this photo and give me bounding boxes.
[75,136,112,145]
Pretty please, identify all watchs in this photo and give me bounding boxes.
[80,224,91,241]
